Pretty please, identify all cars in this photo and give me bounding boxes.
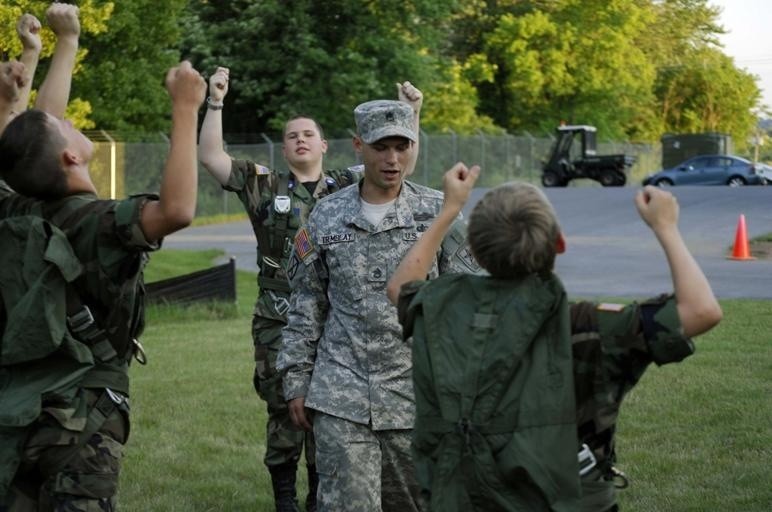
[642,153,765,189]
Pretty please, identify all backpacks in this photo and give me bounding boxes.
[0,193,129,431]
[407,273,618,512]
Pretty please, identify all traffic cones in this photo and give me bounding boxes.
[723,214,755,259]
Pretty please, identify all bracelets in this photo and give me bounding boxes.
[206,94,224,111]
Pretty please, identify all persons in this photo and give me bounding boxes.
[385,160,727,511]
[274,99,494,511]
[1,52,212,511]
[196,66,427,511]
[12,2,83,126]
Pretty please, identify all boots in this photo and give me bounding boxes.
[306,464,319,512]
[269,465,300,512]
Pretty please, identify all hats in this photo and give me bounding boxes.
[354,99,417,145]
